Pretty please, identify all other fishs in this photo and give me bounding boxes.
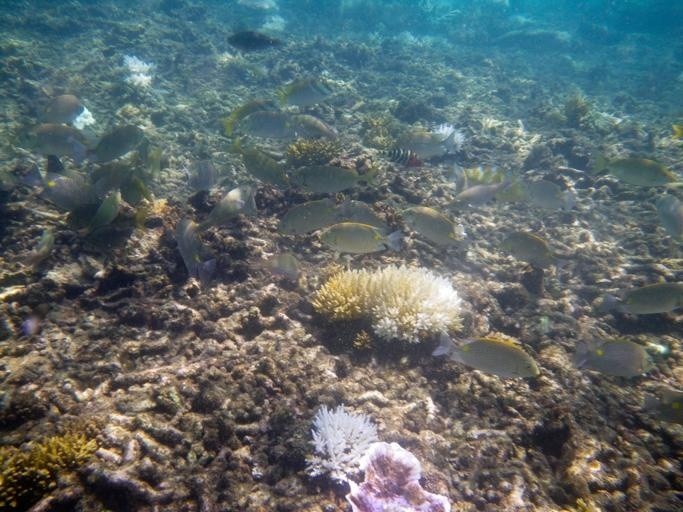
[1,27,682,387]
